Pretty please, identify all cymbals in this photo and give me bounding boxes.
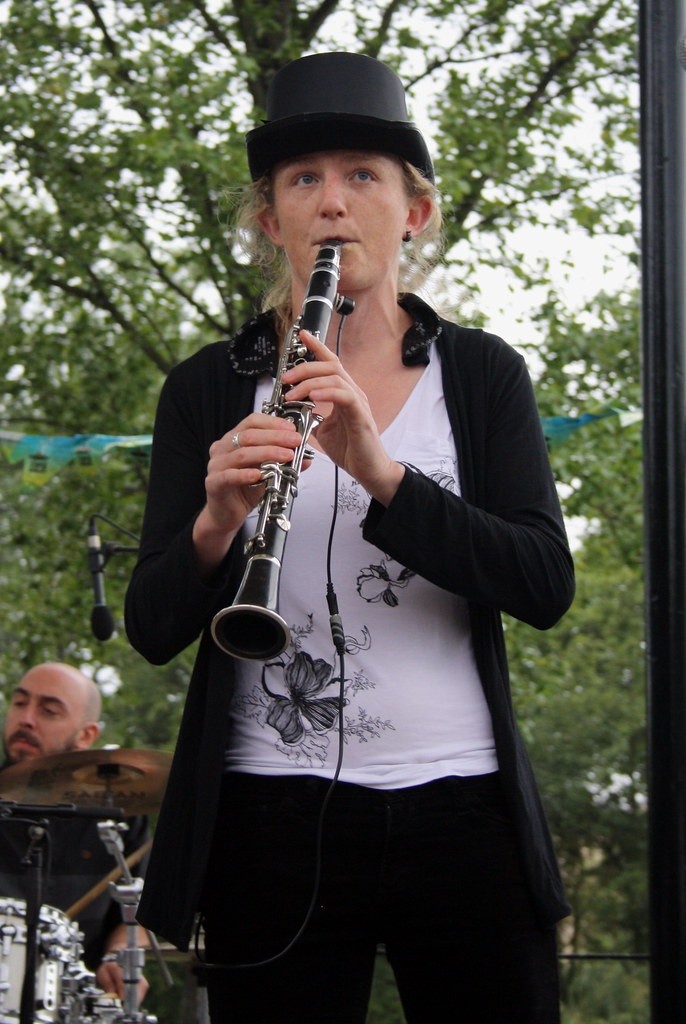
[0,748,174,812]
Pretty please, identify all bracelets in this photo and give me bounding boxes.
[101,952,117,962]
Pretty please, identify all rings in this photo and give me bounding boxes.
[232,432,240,448]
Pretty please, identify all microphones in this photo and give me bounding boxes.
[88,522,113,640]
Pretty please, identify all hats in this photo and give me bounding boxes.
[244,51,434,182]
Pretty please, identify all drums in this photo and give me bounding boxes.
[133,934,209,1024]
[0,897,75,1024]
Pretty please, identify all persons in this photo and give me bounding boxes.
[124,51,577,1024]
[1,661,149,1005]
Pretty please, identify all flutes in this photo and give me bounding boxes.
[209,236,353,665]
